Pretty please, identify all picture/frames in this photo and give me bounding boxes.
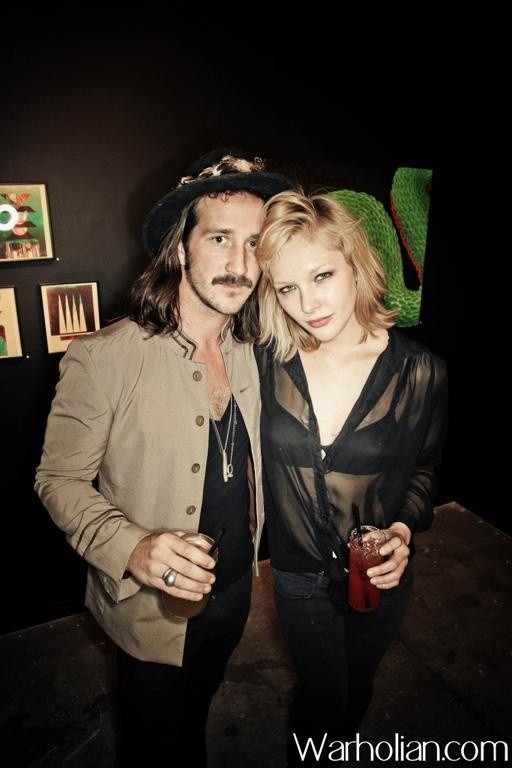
[0,181,55,263]
[0,284,25,360]
[39,280,102,357]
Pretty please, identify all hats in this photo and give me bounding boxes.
[141,144,299,261]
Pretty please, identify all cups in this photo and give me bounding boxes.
[160,531,219,620]
[345,523,385,617]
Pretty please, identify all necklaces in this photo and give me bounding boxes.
[206,389,237,483]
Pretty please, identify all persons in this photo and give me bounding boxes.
[35,190,267,767]
[253,190,449,767]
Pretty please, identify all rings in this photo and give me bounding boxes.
[160,566,176,585]
